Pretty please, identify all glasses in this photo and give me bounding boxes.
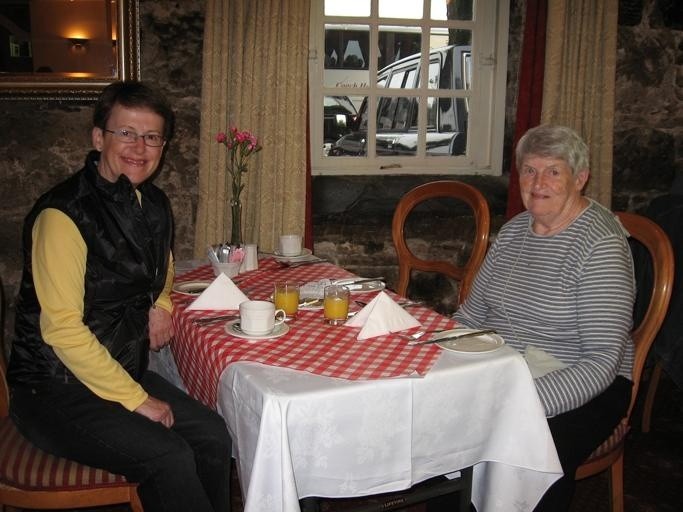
[104,129,166,147]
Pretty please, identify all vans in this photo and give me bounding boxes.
[322,23,422,111]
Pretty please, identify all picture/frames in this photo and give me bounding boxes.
[0,1,144,105]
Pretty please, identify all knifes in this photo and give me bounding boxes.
[345,276,384,287]
[392,330,498,348]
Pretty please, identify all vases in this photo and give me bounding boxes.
[213,123,267,250]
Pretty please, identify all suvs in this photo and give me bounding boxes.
[328,44,471,157]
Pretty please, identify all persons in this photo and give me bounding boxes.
[442,124,639,512]
[5,81,236,511]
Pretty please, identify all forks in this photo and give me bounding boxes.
[385,325,473,342]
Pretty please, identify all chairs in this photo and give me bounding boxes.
[571,208,677,512]
[0,362,142,512]
[390,176,491,314]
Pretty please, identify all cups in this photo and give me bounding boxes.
[273,281,300,320]
[276,234,302,257]
[243,242,258,273]
[237,300,287,335]
[322,284,349,326]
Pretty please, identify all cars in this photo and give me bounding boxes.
[324,97,359,155]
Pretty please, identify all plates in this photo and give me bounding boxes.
[333,278,386,294]
[269,245,312,259]
[223,319,289,342]
[268,293,324,311]
[432,329,504,355]
[169,280,212,296]
